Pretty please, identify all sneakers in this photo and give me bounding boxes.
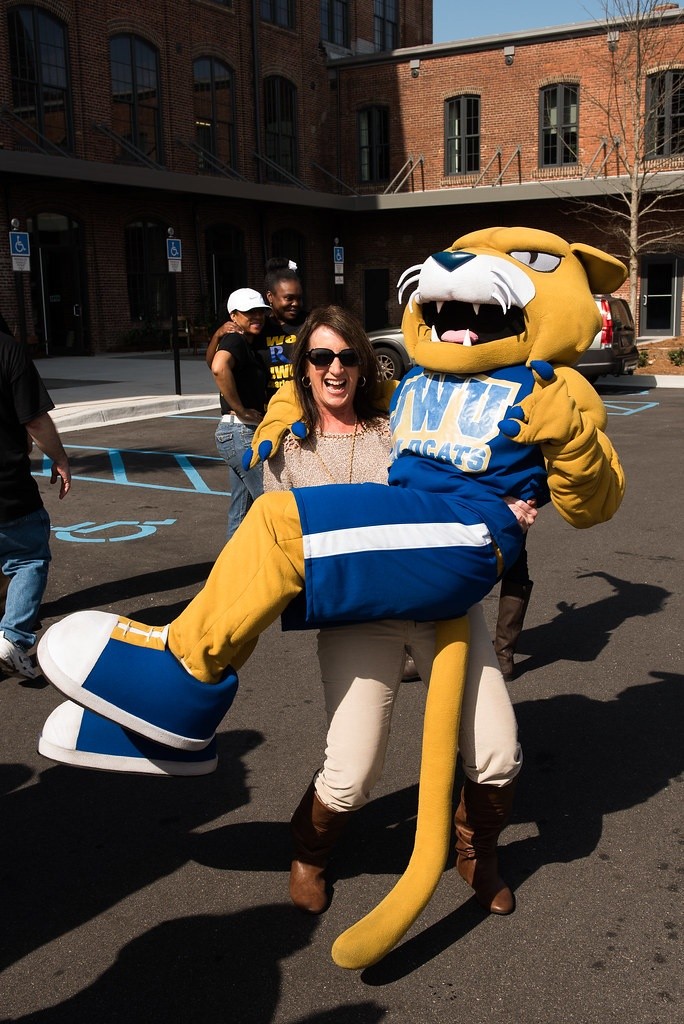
[0,630,36,679]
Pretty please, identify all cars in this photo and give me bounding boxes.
[364,290,641,389]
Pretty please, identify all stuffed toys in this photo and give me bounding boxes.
[36,226,627,777]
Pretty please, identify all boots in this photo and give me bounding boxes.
[289,765,368,913]
[490,578,533,681]
[400,651,420,680]
[452,775,516,914]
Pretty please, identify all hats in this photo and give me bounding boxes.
[228,288,273,315]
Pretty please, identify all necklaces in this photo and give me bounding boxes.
[306,414,358,484]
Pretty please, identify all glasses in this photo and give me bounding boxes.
[305,347,360,367]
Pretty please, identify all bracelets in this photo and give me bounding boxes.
[234,407,242,412]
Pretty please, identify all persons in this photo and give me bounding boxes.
[260,305,534,916]
[1,317,72,677]
[205,260,306,418]
[211,288,277,540]
